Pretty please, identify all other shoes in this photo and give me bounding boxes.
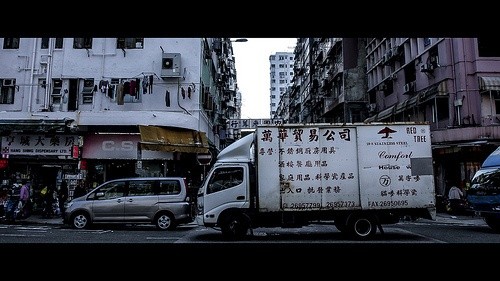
[451,216,456,218]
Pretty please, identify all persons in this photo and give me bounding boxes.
[448,182,464,219]
[73,179,87,199]
[19,178,29,219]
[59,181,69,222]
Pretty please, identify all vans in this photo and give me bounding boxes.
[63,178,191,230]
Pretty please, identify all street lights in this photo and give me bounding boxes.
[218,38,248,122]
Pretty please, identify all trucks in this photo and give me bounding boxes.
[195,122,436,239]
[466,146,500,234]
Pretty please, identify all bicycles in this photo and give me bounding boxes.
[41,201,56,218]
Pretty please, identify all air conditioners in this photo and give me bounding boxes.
[368,104,376,112]
[382,52,389,64]
[420,64,433,71]
[404,83,413,92]
[389,49,397,58]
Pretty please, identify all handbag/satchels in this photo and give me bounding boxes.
[18,201,23,209]
